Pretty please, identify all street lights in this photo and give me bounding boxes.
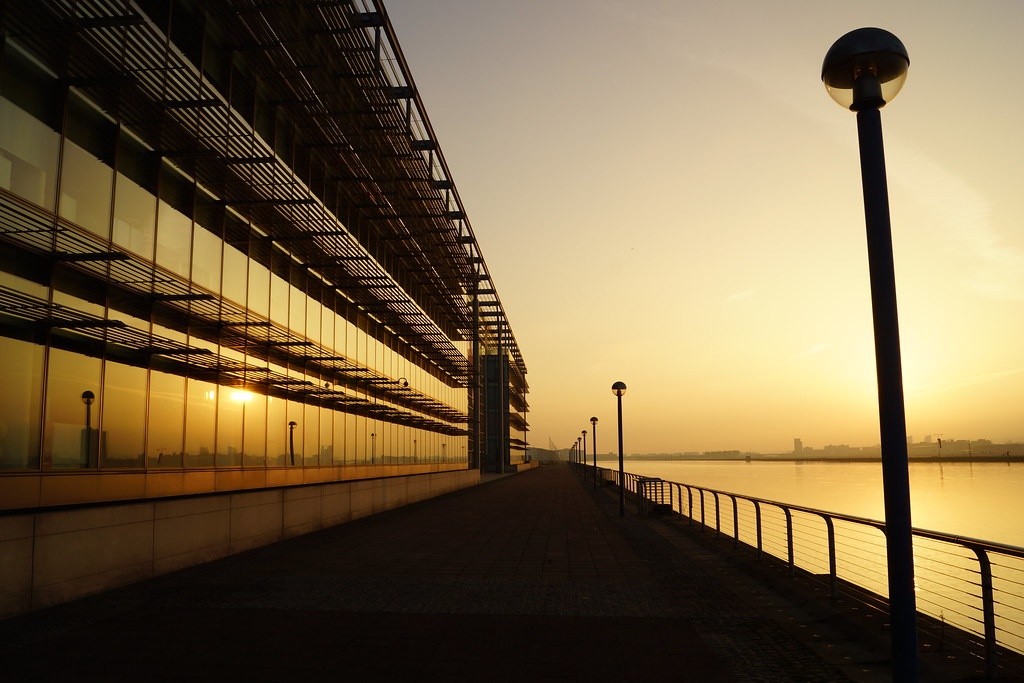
[289,421,297,467]
[568,444,575,466]
[590,417,599,489]
[578,437,581,476]
[413,439,417,465]
[582,430,587,482]
[821,26,922,683]
[81,390,95,469]
[442,444,446,463]
[611,381,628,517]
[371,433,377,466]
